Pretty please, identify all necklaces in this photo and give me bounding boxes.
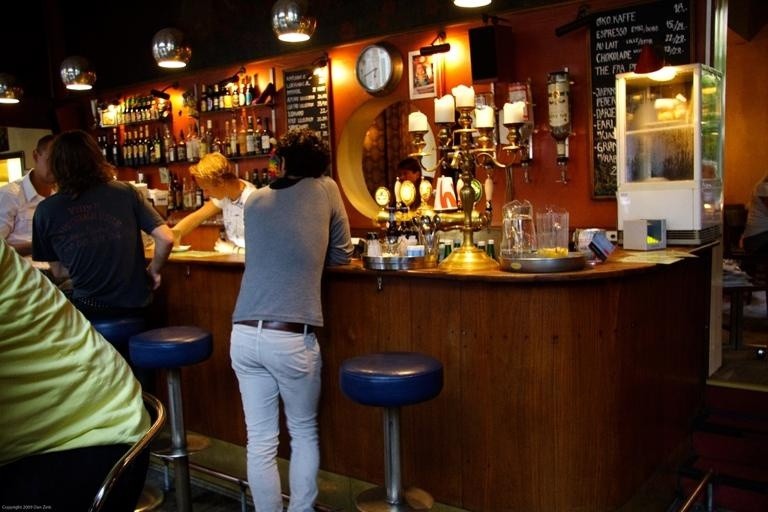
[230,184,245,206]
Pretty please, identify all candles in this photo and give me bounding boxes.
[451,84,475,107]
[503,101,525,124]
[409,110,427,131]
[474,105,493,127]
[434,94,455,122]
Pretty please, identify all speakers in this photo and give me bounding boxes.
[469,22,515,85]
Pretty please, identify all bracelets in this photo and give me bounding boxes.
[232,245,241,255]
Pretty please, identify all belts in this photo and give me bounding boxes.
[233,318,314,334]
[76,297,111,308]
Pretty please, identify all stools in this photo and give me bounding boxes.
[89,315,141,339]
[129,325,213,512]
[339,351,444,512]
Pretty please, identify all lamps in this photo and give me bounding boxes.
[59,56,97,90]
[420,31,451,55]
[634,1,665,74]
[271,0,316,43]
[152,25,192,68]
[0,76,23,104]
[151,81,178,99]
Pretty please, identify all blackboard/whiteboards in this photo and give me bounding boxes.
[283,58,334,177]
[589,0,695,199]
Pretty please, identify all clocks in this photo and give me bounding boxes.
[356,41,403,96]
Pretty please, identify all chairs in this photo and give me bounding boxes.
[90,393,167,512]
[723,205,768,304]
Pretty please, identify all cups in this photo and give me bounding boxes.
[501,198,571,258]
[577,228,607,265]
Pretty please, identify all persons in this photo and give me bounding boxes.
[170,149,258,255]
[0,238,154,512]
[391,158,433,213]
[30,128,176,325]
[0,133,64,289]
[741,175,768,280]
[224,125,353,511]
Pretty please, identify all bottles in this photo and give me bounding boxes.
[198,75,283,188]
[365,217,496,272]
[509,81,535,164]
[97,94,196,214]
[544,71,572,166]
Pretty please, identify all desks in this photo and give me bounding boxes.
[723,260,768,352]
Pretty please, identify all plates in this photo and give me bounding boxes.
[172,245,191,252]
[498,248,587,273]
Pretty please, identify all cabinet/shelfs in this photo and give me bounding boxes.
[615,64,724,244]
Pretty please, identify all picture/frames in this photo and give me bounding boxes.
[406,50,445,103]
